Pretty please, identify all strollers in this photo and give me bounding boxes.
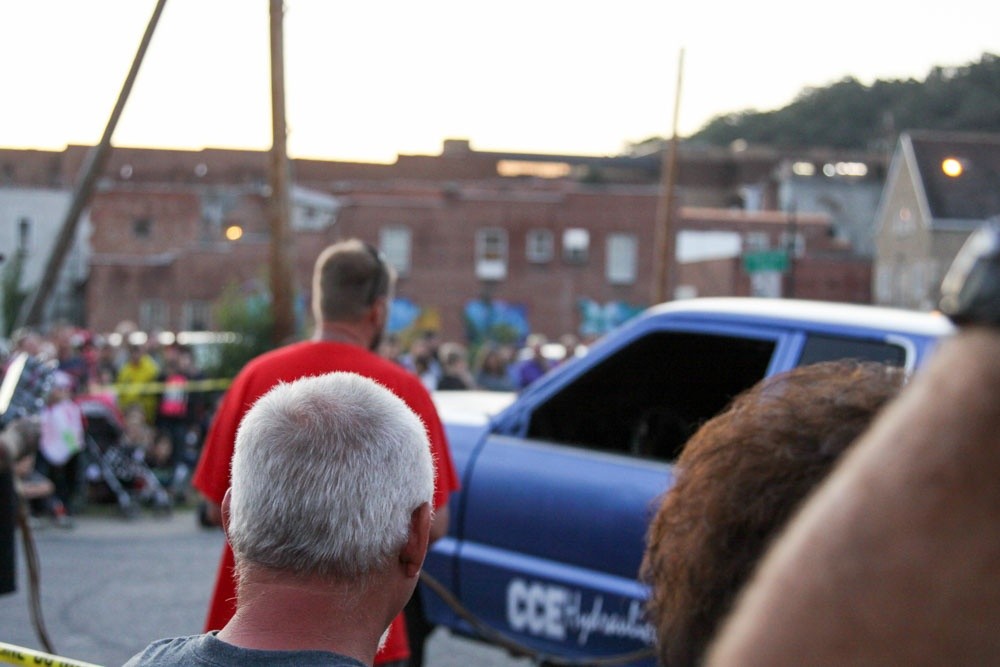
[55,394,180,519]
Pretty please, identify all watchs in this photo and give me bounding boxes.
[939,214,1000,324]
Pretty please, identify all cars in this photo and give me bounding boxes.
[404,296,962,667]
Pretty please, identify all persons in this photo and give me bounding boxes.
[381,329,577,393]
[0,328,210,530]
[119,372,434,667]
[194,239,461,667]
[645,323,1000,667]
